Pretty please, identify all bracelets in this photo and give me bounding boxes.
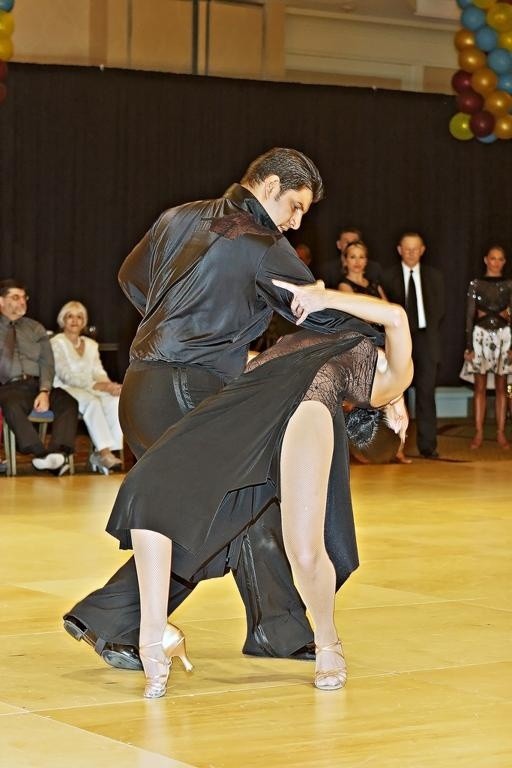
[39,389,50,393]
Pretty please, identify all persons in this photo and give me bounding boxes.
[0,278,80,476]
[458,244,512,453]
[104,276,417,702]
[336,241,412,468]
[322,228,382,289]
[266,245,313,346]
[383,231,449,459]
[49,300,124,476]
[61,146,415,673]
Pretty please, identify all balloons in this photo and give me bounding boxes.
[448,0,512,144]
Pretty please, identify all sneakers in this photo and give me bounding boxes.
[32,452,70,476]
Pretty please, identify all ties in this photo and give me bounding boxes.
[0,321,16,385]
[407,270,418,334]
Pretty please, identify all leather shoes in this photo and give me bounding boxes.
[64,616,143,671]
[470,436,482,449]
[496,435,510,450]
[386,452,412,464]
[290,639,315,660]
[423,448,439,459]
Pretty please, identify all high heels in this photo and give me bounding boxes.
[89,454,122,475]
[314,634,348,690]
[142,622,194,699]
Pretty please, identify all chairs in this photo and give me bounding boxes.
[1,407,81,480]
[76,412,126,472]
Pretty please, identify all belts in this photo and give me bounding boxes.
[8,375,34,384]
